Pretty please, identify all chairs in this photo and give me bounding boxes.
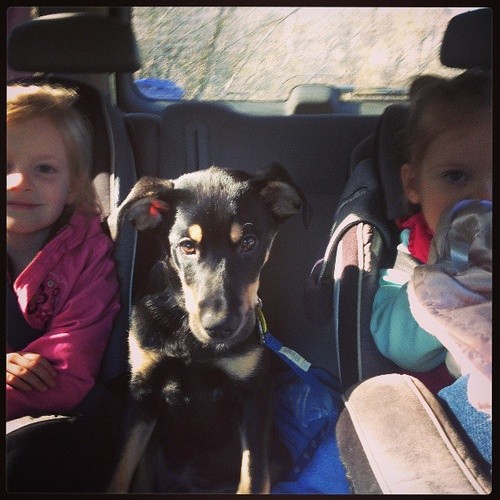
[3,6,144,498]
[330,7,494,400]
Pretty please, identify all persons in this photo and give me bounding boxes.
[368,67,492,483]
[5,83,122,421]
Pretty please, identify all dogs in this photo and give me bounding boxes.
[105,163,313,494]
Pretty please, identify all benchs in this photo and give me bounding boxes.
[121,96,384,476]
